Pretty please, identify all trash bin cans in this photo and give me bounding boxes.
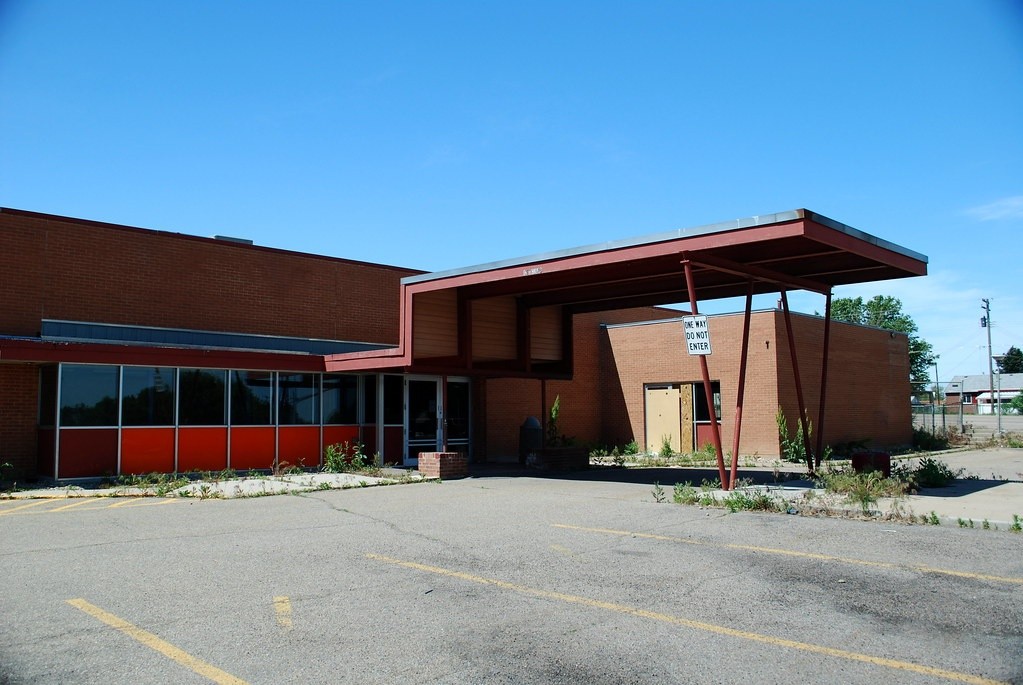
[519,416,542,464]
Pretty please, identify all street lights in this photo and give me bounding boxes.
[926,362,940,406]
[993,355,1005,435]
